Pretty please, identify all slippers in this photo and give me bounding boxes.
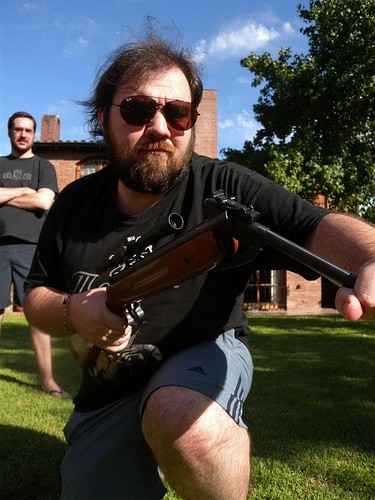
[48,386,69,398]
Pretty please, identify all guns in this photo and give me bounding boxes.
[64,191,358,372]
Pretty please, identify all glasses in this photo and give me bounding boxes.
[111,94,199,131]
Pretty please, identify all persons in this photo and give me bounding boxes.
[23,5,375,499]
[0,111,72,399]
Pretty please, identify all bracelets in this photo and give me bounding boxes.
[61,293,76,334]
